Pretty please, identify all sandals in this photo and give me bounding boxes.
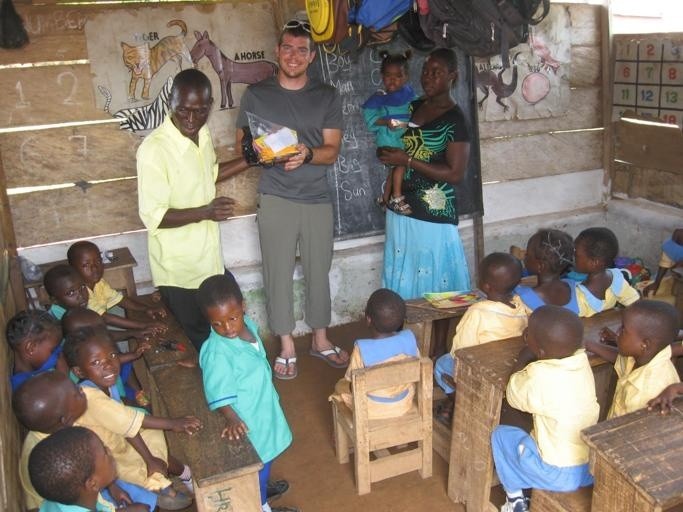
[434,404,451,424]
[387,193,413,216]
[374,196,389,215]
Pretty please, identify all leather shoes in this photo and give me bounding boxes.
[270,506,298,512]
[265,480,289,498]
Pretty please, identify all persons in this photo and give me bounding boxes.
[490,305,600,512]
[237,21,352,380]
[511,228,579,318]
[64,324,203,511]
[643,230,683,314]
[43,264,168,348]
[199,275,300,512]
[61,307,158,413]
[28,426,151,512]
[329,288,422,421]
[136,69,251,352]
[377,48,471,301]
[5,309,65,392]
[434,252,534,419]
[574,228,640,317]
[584,299,683,420]
[12,371,158,512]
[67,241,168,333]
[363,54,426,215]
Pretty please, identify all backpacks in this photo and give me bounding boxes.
[350,0,412,31]
[413,0,549,69]
[302,0,352,45]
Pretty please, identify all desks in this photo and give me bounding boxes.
[117,290,265,512]
[403,275,538,359]
[581,399,683,512]
[24,246,138,310]
[448,303,625,511]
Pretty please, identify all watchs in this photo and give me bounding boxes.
[304,146,313,163]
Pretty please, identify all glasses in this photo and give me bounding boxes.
[282,19,311,33]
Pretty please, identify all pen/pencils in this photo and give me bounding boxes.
[405,303,455,313]
[419,290,481,306]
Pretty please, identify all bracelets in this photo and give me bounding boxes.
[407,156,412,169]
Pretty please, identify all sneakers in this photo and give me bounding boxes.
[500,495,531,512]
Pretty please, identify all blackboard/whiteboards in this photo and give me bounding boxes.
[306,31,484,242]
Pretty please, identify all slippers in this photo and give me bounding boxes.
[273,356,298,380]
[308,344,351,369]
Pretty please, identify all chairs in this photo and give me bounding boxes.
[330,357,435,498]
